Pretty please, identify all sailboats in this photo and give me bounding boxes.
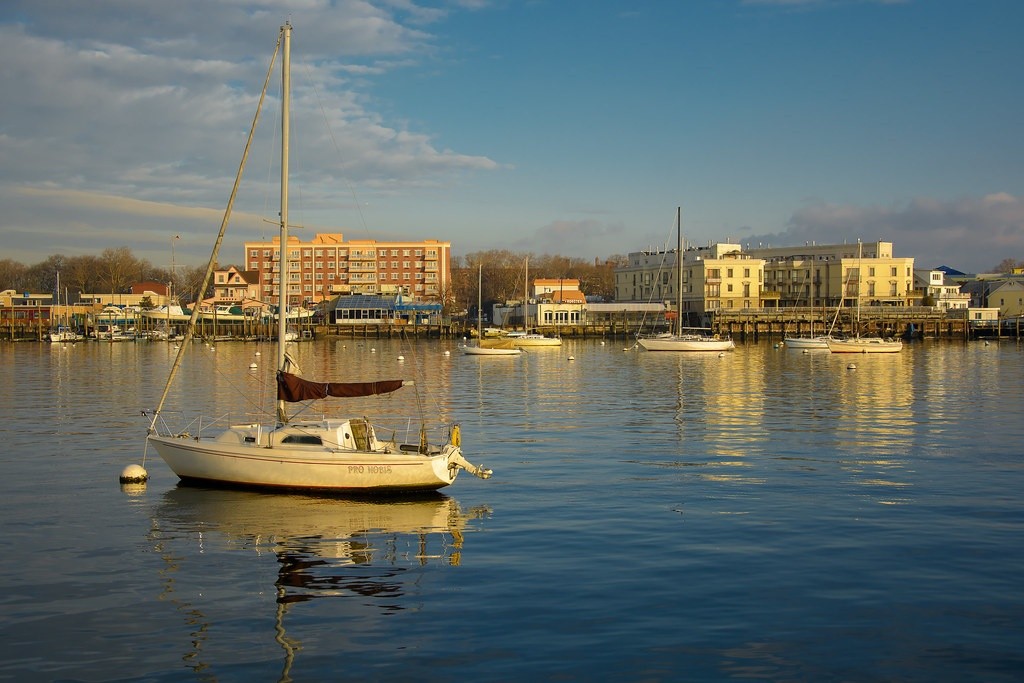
[144,20,494,494]
[636,207,736,351]
[458,262,526,355]
[824,241,904,354]
[783,259,841,350]
[497,257,563,346]
